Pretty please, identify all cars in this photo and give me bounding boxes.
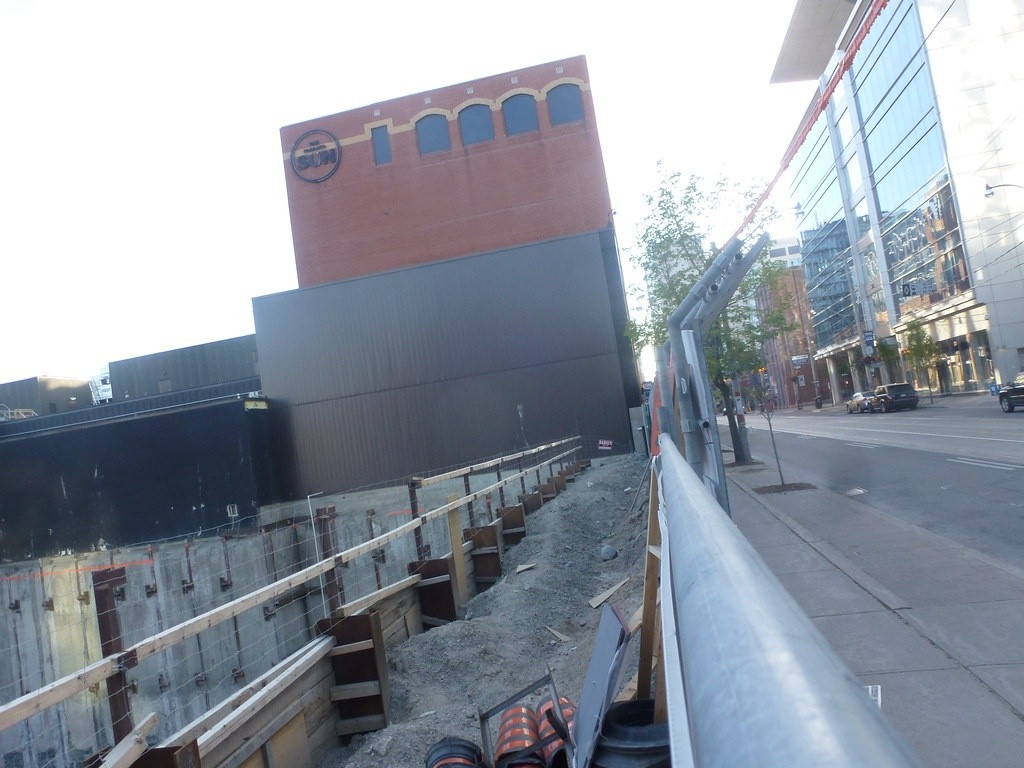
[722,408,727,415]
[998,371,1024,413]
[733,406,746,415]
[846,390,874,414]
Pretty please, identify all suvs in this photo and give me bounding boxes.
[867,381,920,413]
